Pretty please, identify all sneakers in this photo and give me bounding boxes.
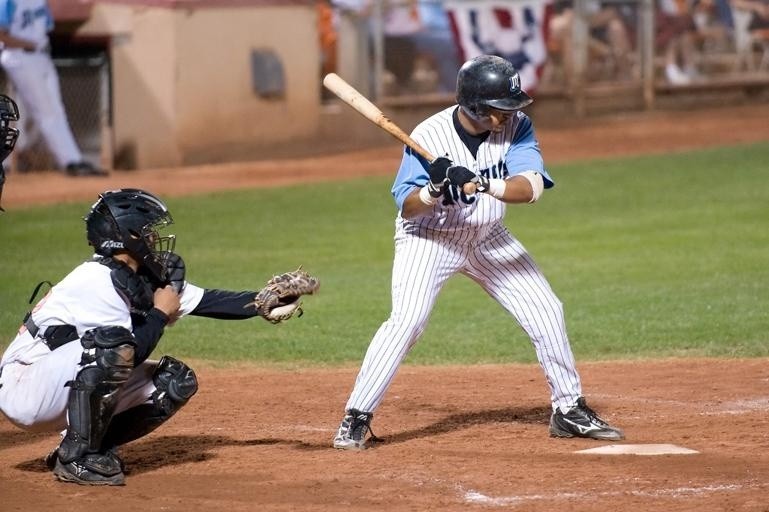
[333,409,373,449]
[549,397,625,441]
[45,446,125,486]
[67,162,104,174]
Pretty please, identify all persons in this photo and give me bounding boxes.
[0,0,113,179]
[0,188,320,486]
[0,94,21,206]
[316,1,769,102]
[335,54,627,448]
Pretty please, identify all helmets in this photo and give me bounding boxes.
[456,54,533,122]
[81,188,176,264]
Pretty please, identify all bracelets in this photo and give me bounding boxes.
[487,177,506,200]
[420,185,438,208]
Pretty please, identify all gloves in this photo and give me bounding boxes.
[447,165,489,194]
[428,157,450,197]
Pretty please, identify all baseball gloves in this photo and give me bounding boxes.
[244,265,320,324]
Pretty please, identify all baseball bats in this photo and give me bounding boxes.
[323,72,476,195]
